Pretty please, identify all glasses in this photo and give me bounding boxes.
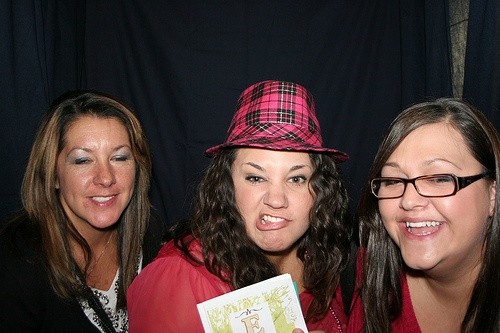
[370,172,486,199]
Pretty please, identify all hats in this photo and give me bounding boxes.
[206,80,349,163]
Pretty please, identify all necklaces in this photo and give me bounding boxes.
[88,231,114,272]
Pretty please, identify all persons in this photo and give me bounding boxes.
[126,80,367,333]
[0,90,165,333]
[348,98,500,333]
